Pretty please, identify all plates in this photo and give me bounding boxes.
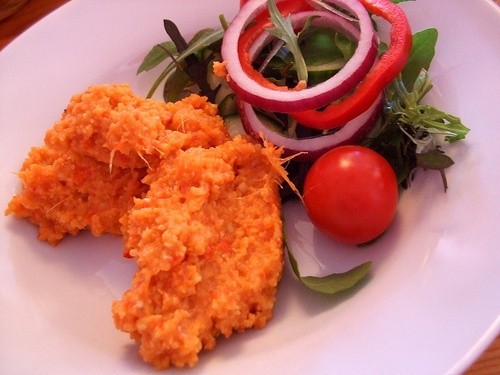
[2,2,500,374]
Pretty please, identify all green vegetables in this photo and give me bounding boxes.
[136,0,470,296]
